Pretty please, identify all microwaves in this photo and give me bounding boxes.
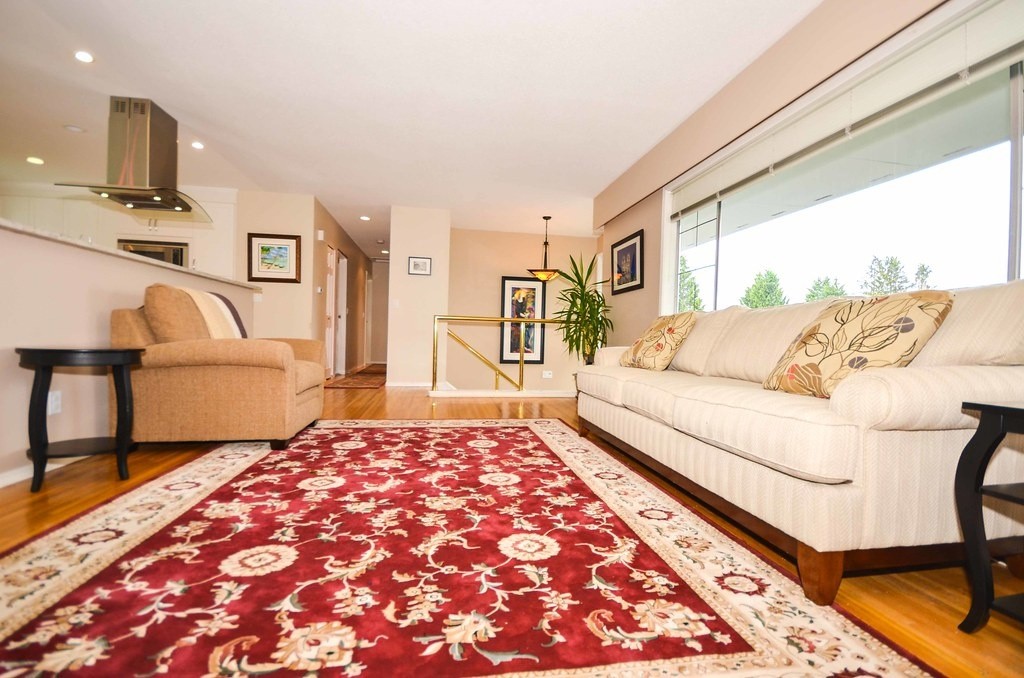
[117,239,189,270]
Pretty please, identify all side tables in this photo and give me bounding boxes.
[954,401,1024,633]
[15,346,146,493]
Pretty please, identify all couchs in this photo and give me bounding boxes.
[576,282,1024,606]
[109,282,327,451]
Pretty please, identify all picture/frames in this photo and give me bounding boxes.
[611,228,644,296]
[247,232,301,283]
[408,256,431,276]
[500,276,546,364]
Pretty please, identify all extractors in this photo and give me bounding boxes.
[53,96,213,225]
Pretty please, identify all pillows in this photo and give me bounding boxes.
[763,291,956,399]
[702,294,835,384]
[666,304,739,375]
[618,309,700,371]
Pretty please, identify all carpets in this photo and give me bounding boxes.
[0,417,947,678]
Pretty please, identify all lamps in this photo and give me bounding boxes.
[526,216,562,281]
